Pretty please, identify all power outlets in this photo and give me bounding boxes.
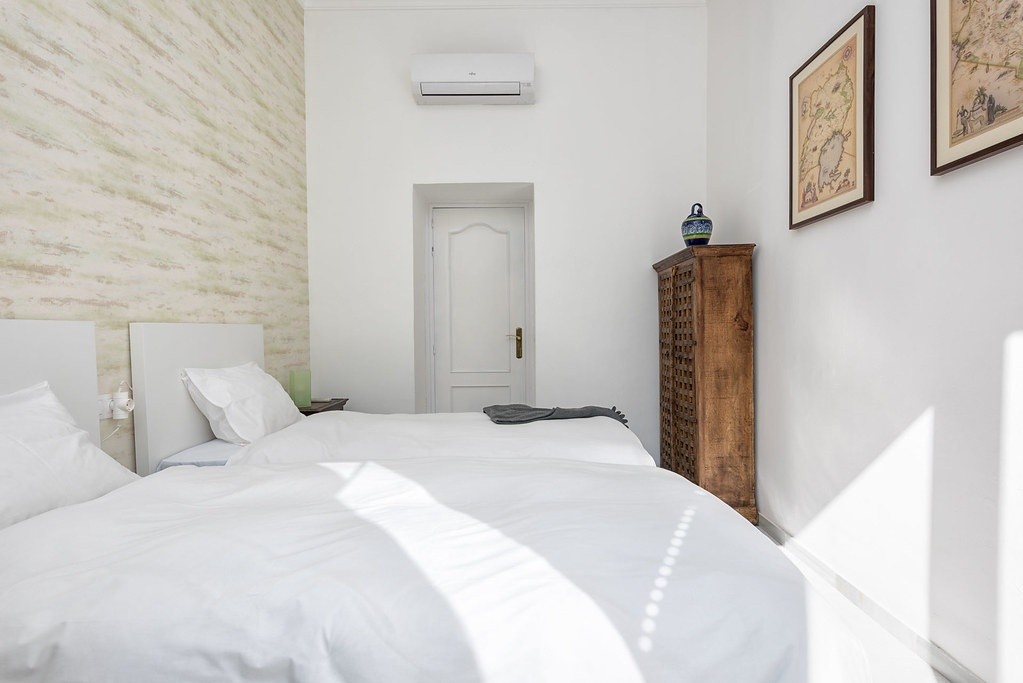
[98,393,113,419]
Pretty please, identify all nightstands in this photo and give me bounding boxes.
[299,398,349,416]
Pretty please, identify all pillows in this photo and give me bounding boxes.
[181,361,306,446]
[1,381,143,528]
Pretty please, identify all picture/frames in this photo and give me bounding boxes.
[931,0,1023,175]
[789,4,876,230]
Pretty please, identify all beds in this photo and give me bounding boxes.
[129,323,657,477]
[2,316,936,683]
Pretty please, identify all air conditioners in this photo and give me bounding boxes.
[411,53,536,105]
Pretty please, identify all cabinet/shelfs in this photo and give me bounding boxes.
[652,243,758,527]
